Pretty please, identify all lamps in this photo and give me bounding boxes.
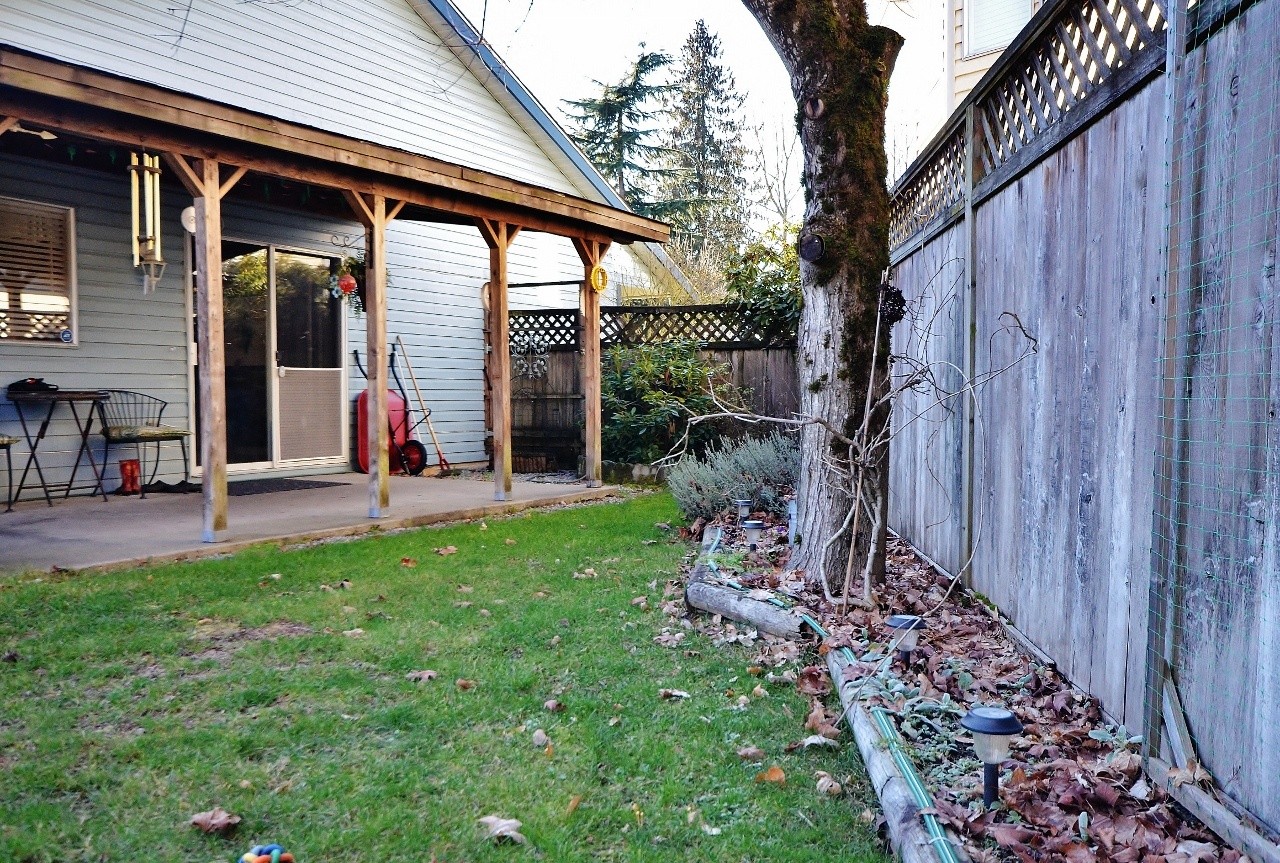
[960,706,1026,767]
[734,499,752,518]
[740,521,765,544]
[883,615,927,653]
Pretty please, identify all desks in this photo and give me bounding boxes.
[5,390,111,506]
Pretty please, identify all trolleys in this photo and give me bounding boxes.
[353,349,427,476]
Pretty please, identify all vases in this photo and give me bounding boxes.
[356,280,366,314]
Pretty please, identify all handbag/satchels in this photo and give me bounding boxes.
[7,377,58,393]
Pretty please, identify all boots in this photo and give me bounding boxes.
[115,459,131,495]
[130,458,140,495]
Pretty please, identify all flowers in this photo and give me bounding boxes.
[328,254,389,312]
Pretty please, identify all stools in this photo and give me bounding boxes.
[0,432,18,513]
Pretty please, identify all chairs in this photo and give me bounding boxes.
[91,390,190,499]
